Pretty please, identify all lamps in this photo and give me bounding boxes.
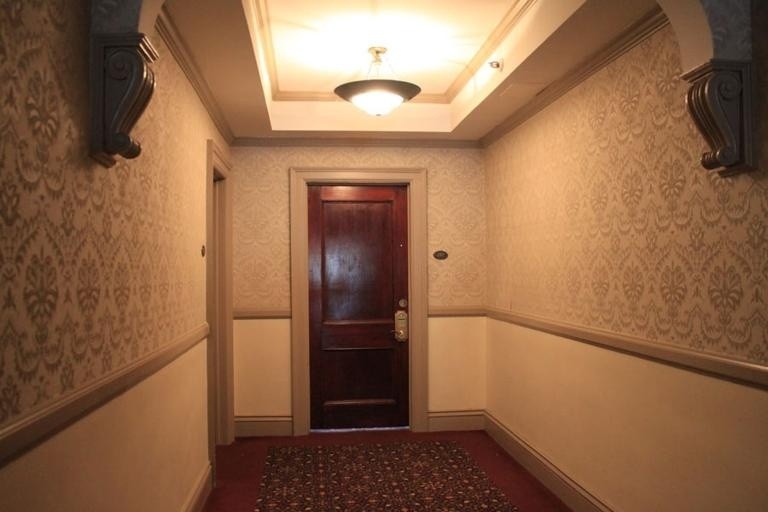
[334,45,421,118]
